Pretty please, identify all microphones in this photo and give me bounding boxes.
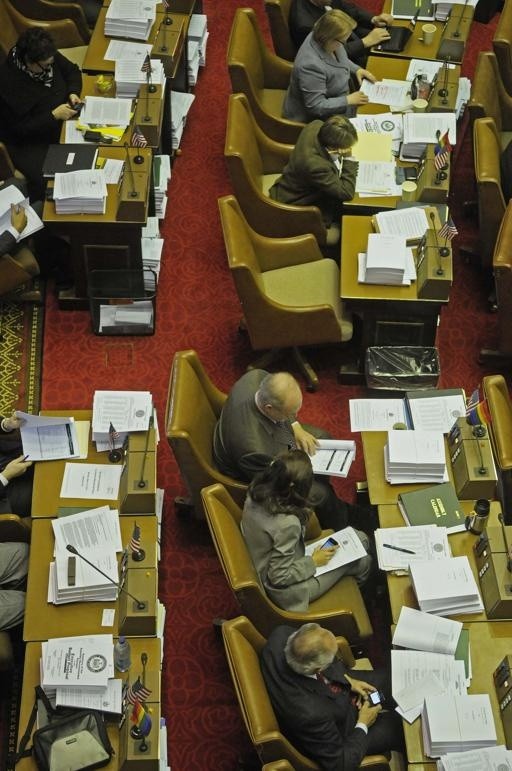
[431,129,444,187]
[133,652,152,755]
[498,512,512,596]
[133,415,154,490]
[123,141,140,199]
[451,0,469,40]
[66,544,149,612]
[159,15,170,54]
[429,212,446,278]
[468,415,489,478]
[141,71,153,123]
[439,61,450,105]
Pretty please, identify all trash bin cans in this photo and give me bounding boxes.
[365,345,440,398]
[86,268,157,336]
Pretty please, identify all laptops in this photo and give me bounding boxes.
[374,7,421,53]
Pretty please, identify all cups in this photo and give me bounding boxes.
[413,98,428,113]
[422,23,437,44]
[95,72,113,95]
[401,181,417,202]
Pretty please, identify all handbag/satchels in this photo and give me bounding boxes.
[31,709,115,771]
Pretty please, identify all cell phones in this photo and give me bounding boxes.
[319,537,339,551]
[70,102,84,111]
[360,689,386,708]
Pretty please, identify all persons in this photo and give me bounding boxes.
[0,180,70,290]
[209,368,335,485]
[281,9,377,125]
[267,113,360,231]
[0,26,82,176]
[236,448,386,616]
[257,620,407,771]
[287,0,395,60]
[0,539,31,634]
[0,410,33,519]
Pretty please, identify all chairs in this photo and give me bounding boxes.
[165,0,512,771]
[0,511,30,676]
[0,0,93,290]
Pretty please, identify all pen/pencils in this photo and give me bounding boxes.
[383,543,415,554]
[21,455,29,462]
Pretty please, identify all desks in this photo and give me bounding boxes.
[14,408,161,771]
[41,0,202,312]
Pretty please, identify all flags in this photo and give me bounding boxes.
[130,522,142,557]
[128,125,148,149]
[161,1,170,11]
[436,213,460,242]
[108,422,120,453]
[465,385,491,428]
[434,128,453,172]
[141,54,154,76]
[126,674,151,734]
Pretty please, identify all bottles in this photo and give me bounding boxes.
[113,635,133,675]
[464,498,491,536]
